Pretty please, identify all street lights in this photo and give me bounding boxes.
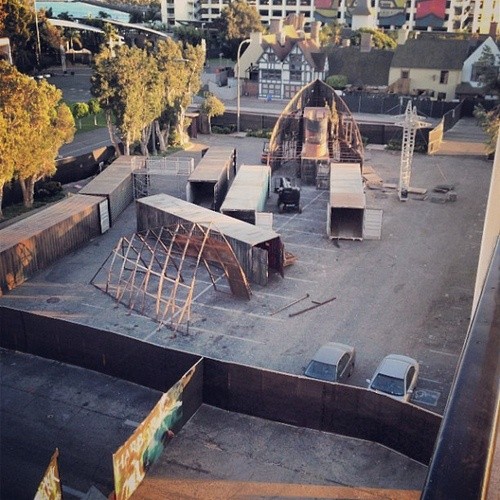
[235,37,254,130]
[202,22,206,30]
